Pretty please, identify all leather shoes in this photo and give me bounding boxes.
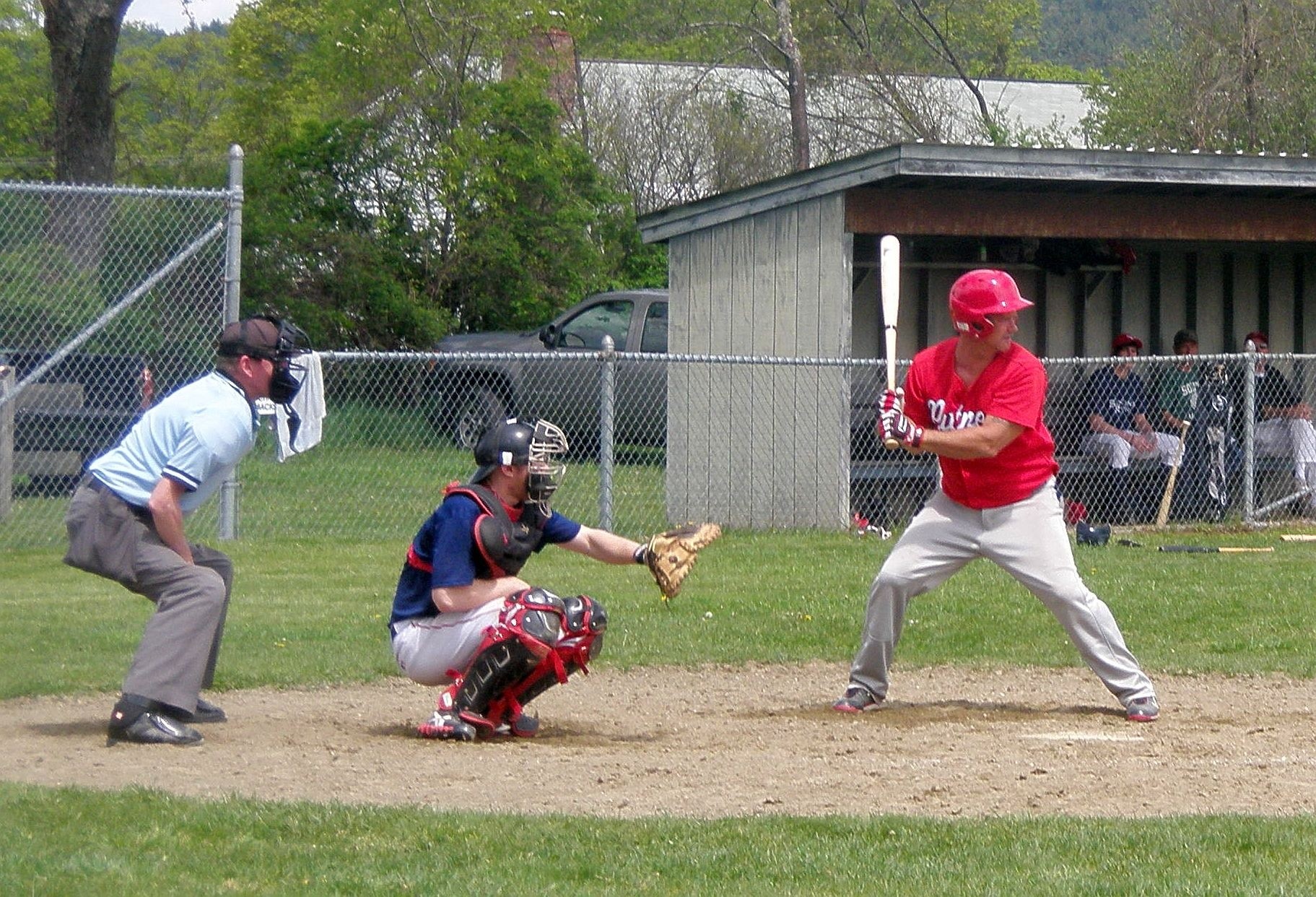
[163,694,228,723]
[106,702,205,747]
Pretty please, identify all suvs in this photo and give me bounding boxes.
[419,288,668,454]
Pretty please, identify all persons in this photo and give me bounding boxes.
[387,416,720,743]
[1077,331,1316,517]
[66,315,313,744]
[832,270,1162,723]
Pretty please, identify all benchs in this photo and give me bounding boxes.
[847,384,1295,521]
[0,348,147,478]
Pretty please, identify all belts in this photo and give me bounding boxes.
[85,476,146,517]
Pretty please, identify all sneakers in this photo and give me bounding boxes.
[833,688,888,713]
[1125,695,1159,721]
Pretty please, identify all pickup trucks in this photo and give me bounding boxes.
[1,350,156,481]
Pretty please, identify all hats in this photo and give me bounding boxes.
[1112,333,1142,352]
[1174,329,1199,348]
[1243,331,1268,344]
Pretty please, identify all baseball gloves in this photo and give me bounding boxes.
[647,523,722,599]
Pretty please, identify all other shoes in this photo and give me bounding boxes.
[416,706,477,741]
[1285,478,1310,496]
[1290,502,1316,519]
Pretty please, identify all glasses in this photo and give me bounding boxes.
[1254,342,1268,351]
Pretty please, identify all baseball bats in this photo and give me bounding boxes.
[1155,420,1191,525]
[1159,546,1275,552]
[879,235,900,450]
[1280,534,1316,541]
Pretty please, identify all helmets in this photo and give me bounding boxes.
[470,419,546,486]
[949,268,1033,344]
[1075,520,1110,547]
[216,314,279,363]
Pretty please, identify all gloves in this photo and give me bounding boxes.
[878,408,924,456]
[875,387,905,419]
